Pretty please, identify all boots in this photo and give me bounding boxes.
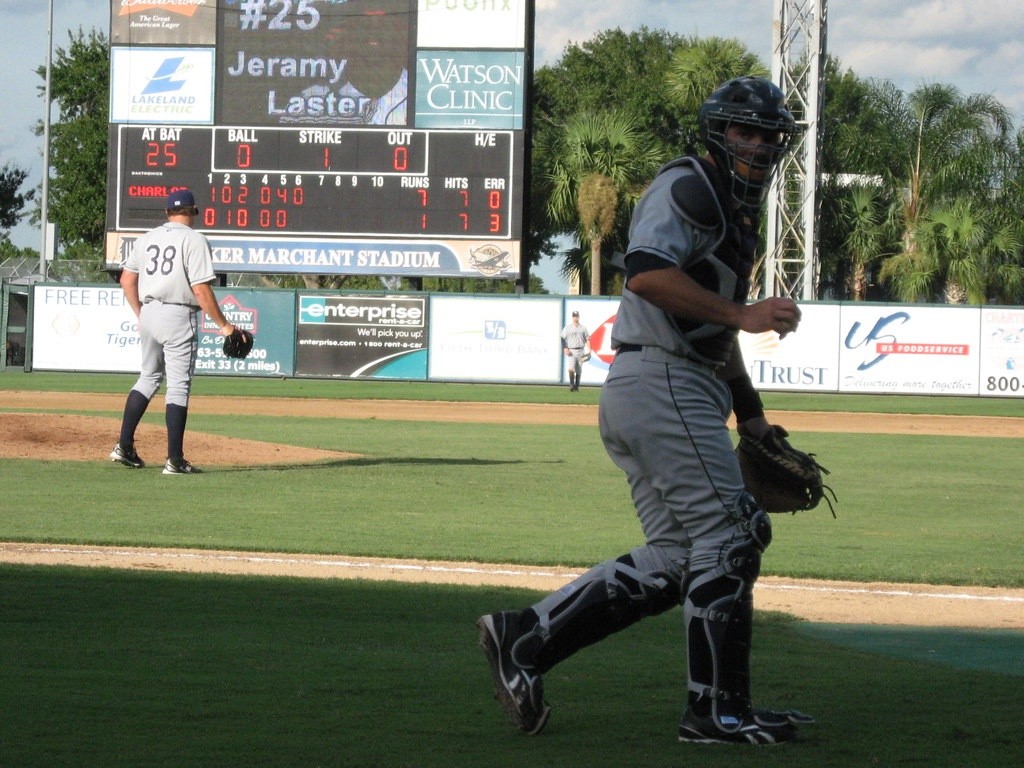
[475,545,682,737]
[676,551,815,748]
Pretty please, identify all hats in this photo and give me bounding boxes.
[164,190,199,217]
[572,311,579,317]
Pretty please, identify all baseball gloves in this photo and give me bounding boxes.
[733,423,826,515]
[221,328,253,360]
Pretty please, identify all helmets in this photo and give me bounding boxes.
[697,75,803,209]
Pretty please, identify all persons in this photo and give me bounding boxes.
[561,311,591,393]
[110,190,253,476]
[477,74,823,745]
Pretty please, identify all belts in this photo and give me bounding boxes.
[614,342,645,355]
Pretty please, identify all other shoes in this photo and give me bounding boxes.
[569,387,580,392]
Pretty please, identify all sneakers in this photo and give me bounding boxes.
[109,443,145,468]
[161,458,203,474]
